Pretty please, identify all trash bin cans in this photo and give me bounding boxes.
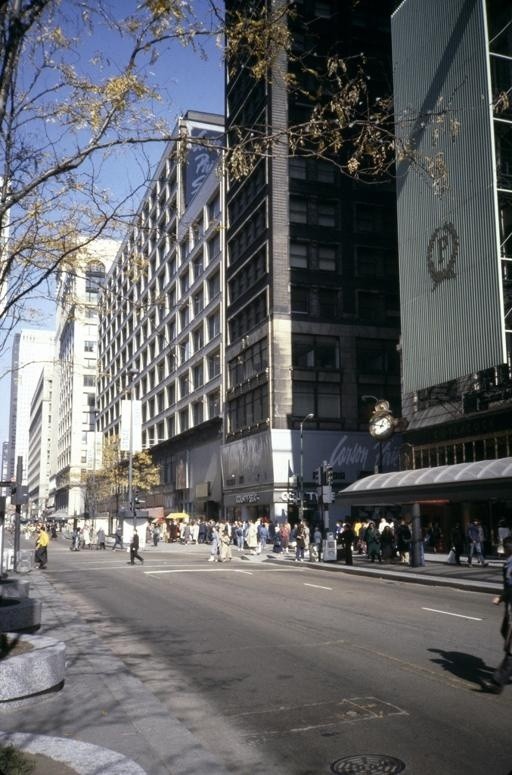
[322,540,337,563]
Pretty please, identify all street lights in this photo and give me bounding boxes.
[359,394,383,471]
[126,365,140,511]
[299,412,316,526]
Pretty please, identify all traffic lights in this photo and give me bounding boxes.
[325,466,333,484]
[312,467,321,486]
[323,486,335,504]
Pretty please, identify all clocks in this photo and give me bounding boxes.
[367,399,410,441]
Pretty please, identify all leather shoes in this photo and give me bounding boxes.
[479,677,501,693]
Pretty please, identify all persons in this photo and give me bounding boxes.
[69,524,124,551]
[146,519,310,562]
[426,517,512,563]
[35,527,50,567]
[335,518,412,565]
[19,523,58,540]
[493,536,512,685]
[127,529,143,565]
